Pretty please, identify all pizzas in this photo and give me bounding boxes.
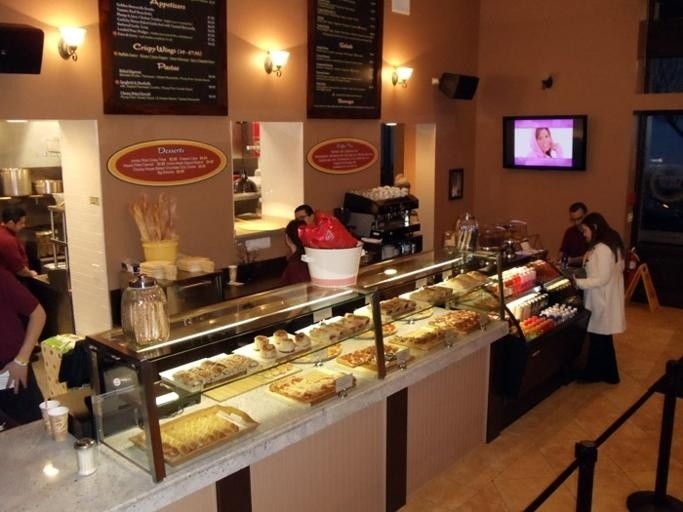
[336,343,400,367]
[431,309,481,333]
[394,324,444,343]
[271,370,357,404]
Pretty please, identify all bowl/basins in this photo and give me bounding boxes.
[0,168,32,197]
[32,180,62,195]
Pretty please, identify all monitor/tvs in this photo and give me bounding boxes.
[502,114,587,172]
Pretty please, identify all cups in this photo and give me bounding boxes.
[349,185,410,199]
[47,407,69,442]
[39,401,59,435]
[228,265,237,283]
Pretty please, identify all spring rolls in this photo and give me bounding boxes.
[311,314,370,342]
[380,297,417,318]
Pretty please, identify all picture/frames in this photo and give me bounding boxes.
[447,167,463,200]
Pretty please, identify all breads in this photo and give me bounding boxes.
[173,353,259,391]
[410,286,453,303]
[161,413,239,457]
[447,271,487,291]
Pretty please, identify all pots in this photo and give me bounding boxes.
[478,224,508,251]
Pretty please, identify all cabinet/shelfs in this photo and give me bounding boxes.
[447,244,592,442]
[358,236,503,382]
[83,279,376,482]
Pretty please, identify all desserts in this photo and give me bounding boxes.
[254,329,312,358]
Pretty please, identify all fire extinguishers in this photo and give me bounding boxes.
[626,247,640,288]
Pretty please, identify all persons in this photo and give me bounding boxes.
[0,268,49,424]
[559,201,590,268]
[569,212,626,385]
[525,128,562,158]
[278,204,314,284]
[0,206,32,279]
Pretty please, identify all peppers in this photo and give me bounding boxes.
[297,210,358,249]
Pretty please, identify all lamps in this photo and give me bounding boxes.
[391,65,413,89]
[263,49,290,78]
[58,26,87,62]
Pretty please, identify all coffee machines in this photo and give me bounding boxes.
[333,192,423,262]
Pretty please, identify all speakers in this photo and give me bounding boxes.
[438,71,479,100]
[0,23,44,74]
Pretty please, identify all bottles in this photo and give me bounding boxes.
[455,211,478,253]
[442,230,455,247]
[561,252,569,270]
[121,274,170,346]
[73,438,96,477]
[486,260,576,342]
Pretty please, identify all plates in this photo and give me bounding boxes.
[288,342,342,363]
[353,324,398,340]
[395,309,433,321]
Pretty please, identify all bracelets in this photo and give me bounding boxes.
[15,356,30,366]
